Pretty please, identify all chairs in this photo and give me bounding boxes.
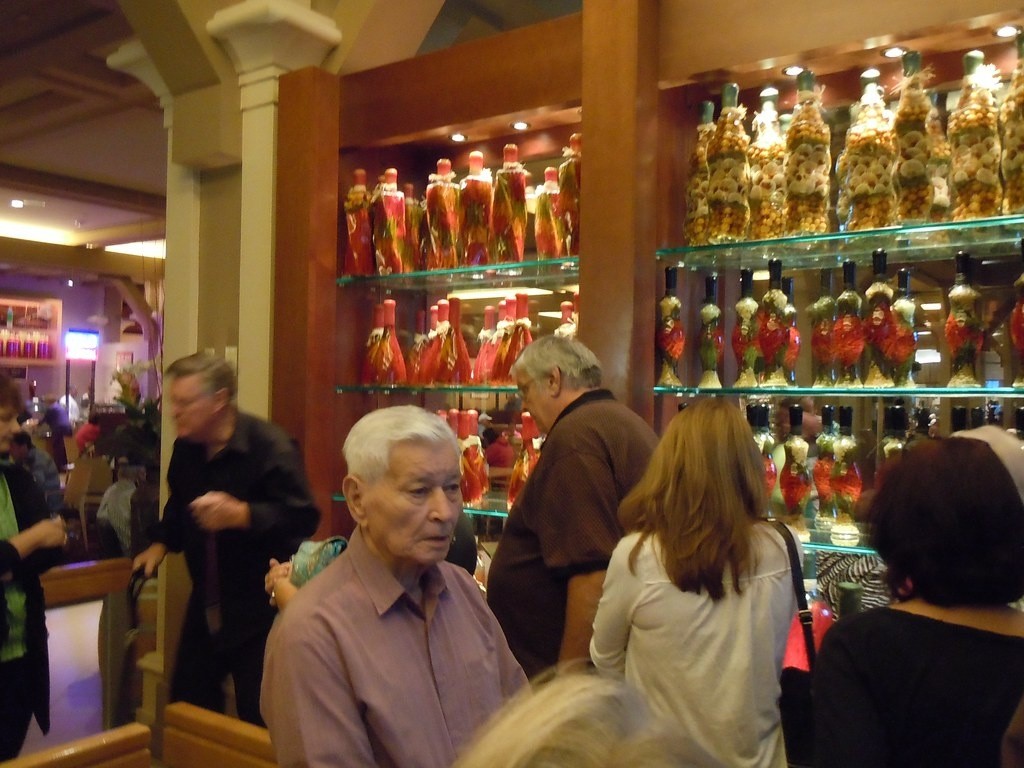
[44,434,117,556]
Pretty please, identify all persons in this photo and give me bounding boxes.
[97,451,147,558]
[485,334,660,688]
[450,659,730,767]
[587,396,802,768]
[0,371,66,762]
[809,424,1024,768]
[75,412,101,456]
[129,352,322,730]
[265,406,535,768]
[60,385,80,425]
[37,394,74,469]
[12,430,60,518]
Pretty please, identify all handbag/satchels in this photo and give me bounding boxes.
[779,665,815,766]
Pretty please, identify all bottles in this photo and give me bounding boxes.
[342,114,580,511]
[658,30,1023,549]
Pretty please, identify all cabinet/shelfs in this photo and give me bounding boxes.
[275,2,1024,619]
[0,295,66,368]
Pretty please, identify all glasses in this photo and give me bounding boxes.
[513,374,543,401]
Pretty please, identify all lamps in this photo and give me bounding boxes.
[448,132,469,143]
[781,64,807,77]
[758,82,780,98]
[856,66,881,79]
[964,47,987,61]
[509,119,529,131]
[880,46,907,57]
[994,23,1018,39]
